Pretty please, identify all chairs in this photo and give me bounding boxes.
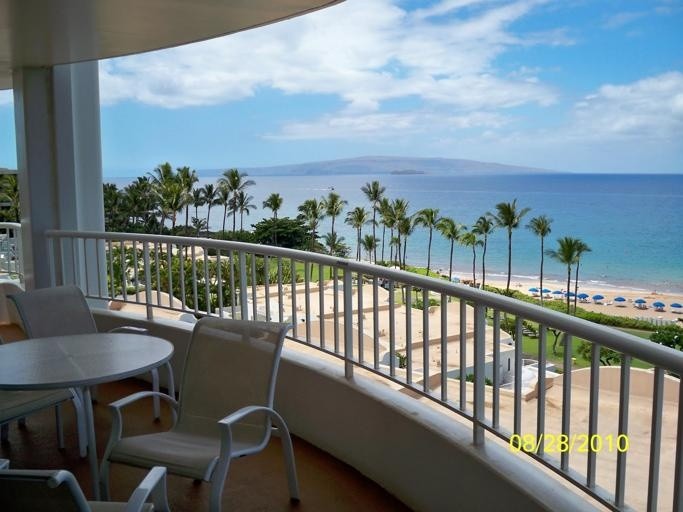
[2,454,170,510]
[96,315,301,509]
[1,389,74,450]
[5,284,162,453]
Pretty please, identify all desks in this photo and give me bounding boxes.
[1,332,179,501]
